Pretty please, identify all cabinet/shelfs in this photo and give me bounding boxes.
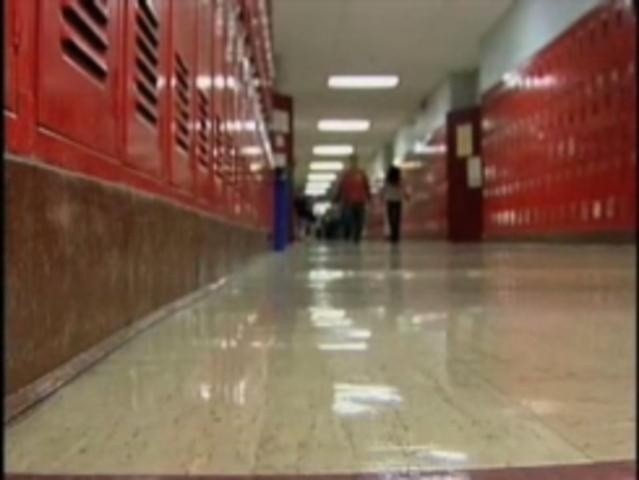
[397,108,480,241]
[478,0,639,239]
[0,0,278,230]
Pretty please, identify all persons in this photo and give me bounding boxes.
[293,151,410,242]
